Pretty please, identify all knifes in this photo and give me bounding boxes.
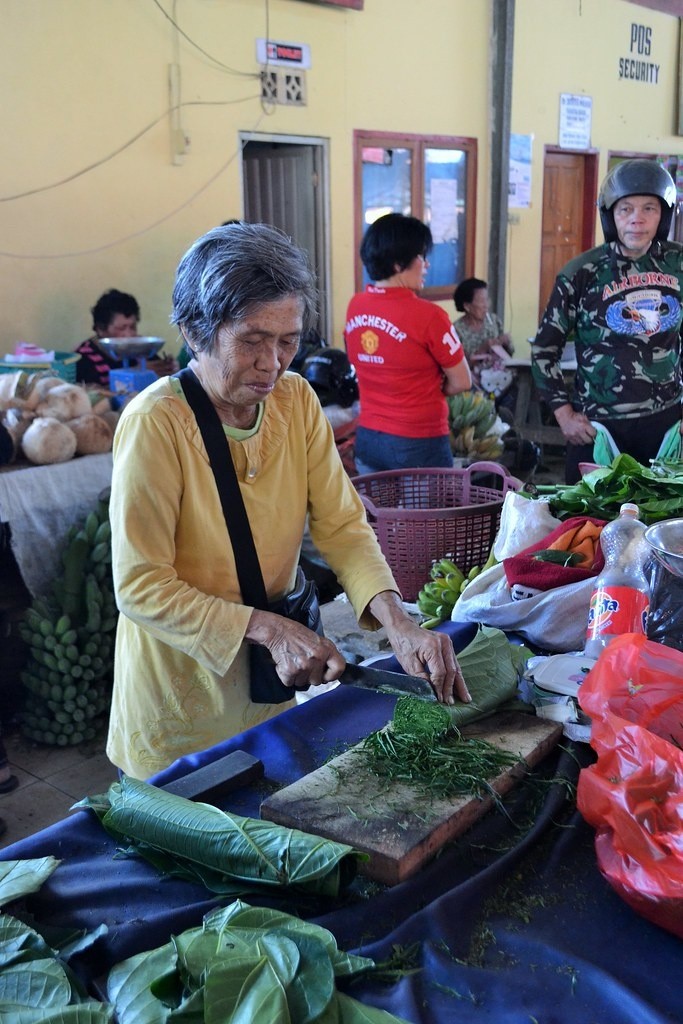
[331,660,439,704]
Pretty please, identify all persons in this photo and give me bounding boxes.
[452,277,515,386]
[77,289,179,410]
[107,224,472,779]
[344,213,473,520]
[531,162,683,485]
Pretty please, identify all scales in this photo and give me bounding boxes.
[96,337,167,408]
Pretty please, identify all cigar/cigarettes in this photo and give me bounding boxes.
[485,312,493,324]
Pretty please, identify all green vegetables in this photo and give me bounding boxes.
[530,449,683,524]
[0,624,552,1023]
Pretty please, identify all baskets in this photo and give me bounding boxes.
[349,459,524,600]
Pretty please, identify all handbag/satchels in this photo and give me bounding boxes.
[247,564,328,705]
[580,629,682,937]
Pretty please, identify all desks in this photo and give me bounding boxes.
[502,357,574,445]
[0,393,358,613]
[0,620,683,1024]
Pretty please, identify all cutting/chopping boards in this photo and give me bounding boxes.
[259,703,566,884]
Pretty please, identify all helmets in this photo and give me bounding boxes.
[599,161,676,244]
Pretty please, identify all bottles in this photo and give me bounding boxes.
[585,502,654,657]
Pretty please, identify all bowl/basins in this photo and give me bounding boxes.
[642,517,683,580]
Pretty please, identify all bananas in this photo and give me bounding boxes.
[416,545,496,629]
[445,391,502,461]
[14,509,120,748]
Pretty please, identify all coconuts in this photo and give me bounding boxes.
[0,367,120,464]
